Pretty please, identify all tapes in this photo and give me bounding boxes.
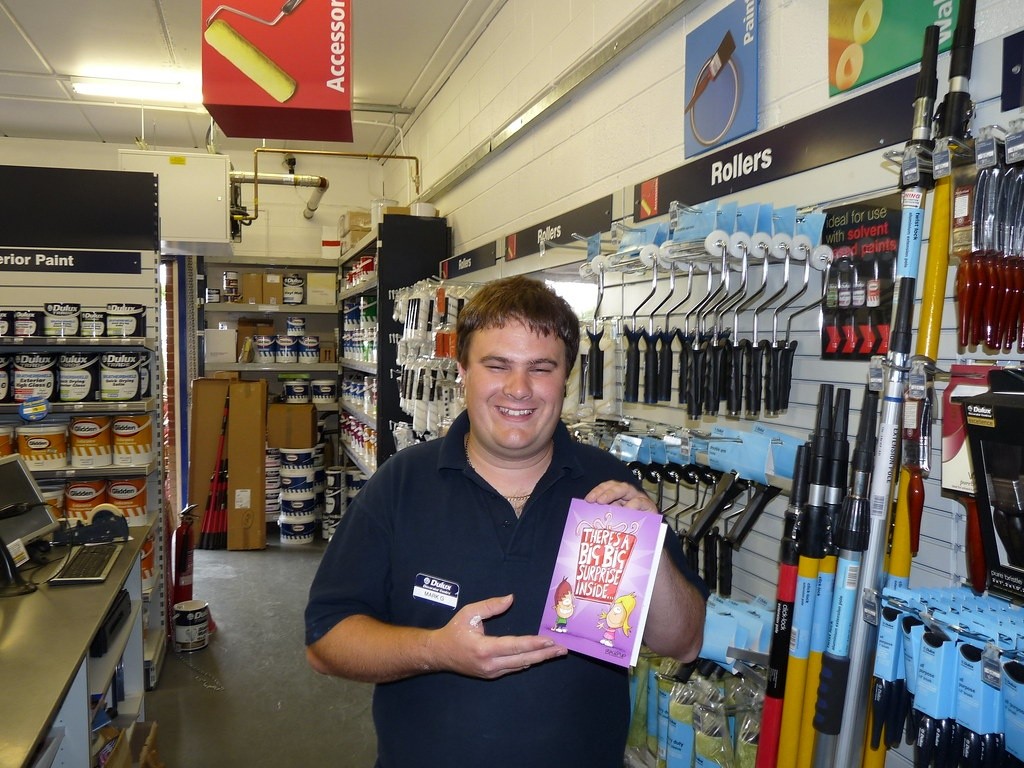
[411,202,437,217]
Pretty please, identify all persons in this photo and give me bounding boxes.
[305,277,711,768]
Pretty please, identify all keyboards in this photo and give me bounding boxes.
[47,544,123,585]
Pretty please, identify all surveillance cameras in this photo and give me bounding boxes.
[284,154,297,166]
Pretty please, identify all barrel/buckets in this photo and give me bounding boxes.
[1,303,155,527]
[172,600,209,653]
[205,256,368,544]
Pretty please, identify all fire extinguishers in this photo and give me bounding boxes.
[174,503,200,603]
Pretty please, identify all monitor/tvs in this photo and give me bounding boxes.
[0,453,60,548]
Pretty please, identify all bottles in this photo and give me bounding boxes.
[340,256,378,475]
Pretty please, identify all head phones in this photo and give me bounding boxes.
[53,504,130,546]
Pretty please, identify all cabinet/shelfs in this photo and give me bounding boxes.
[0,524,151,768]
[339,214,452,479]
[196,255,339,411]
[0,165,169,691]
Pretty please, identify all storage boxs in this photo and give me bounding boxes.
[267,402,318,448]
[102,719,164,768]
[189,372,268,550]
[940,364,1024,608]
[322,240,340,258]
[319,341,335,363]
[237,318,275,360]
[307,272,336,305]
[204,329,236,363]
[244,272,283,304]
[339,207,439,256]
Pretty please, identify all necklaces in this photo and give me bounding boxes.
[466,434,531,500]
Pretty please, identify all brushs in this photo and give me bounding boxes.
[941,391,993,598]
[391,283,469,451]
[869,606,1024,768]
[953,167,1024,355]
[605,231,832,422]
[903,389,933,557]
[982,439,1024,570]
[561,415,805,599]
[684,29,736,114]
[579,255,610,400]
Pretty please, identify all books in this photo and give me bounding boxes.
[538,497,670,670]
